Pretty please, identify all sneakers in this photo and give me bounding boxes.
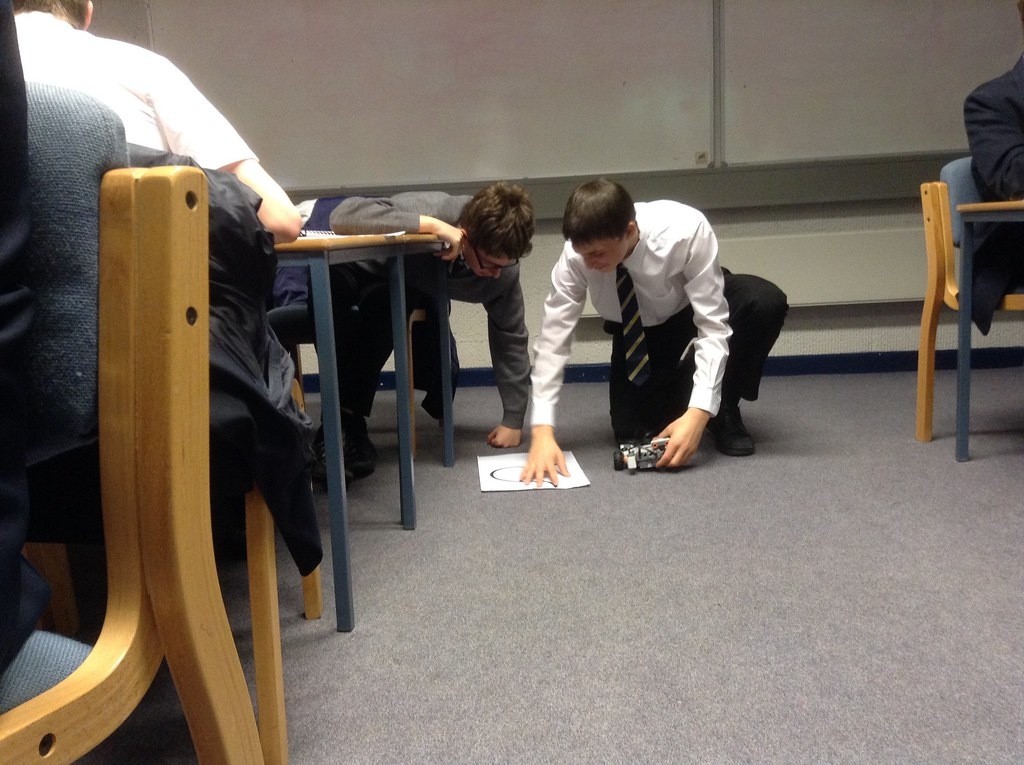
[307,421,376,481]
[705,401,755,457]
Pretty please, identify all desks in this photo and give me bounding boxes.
[269,233,453,633]
[955,200,1024,463]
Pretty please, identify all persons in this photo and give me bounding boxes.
[309,181,537,476]
[519,178,790,488]
[956,1,1024,335]
[0,0,306,765]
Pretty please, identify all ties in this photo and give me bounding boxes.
[615,262,652,387]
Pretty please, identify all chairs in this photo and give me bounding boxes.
[303,195,435,458]
[916,157,1024,442]
[1,84,323,765]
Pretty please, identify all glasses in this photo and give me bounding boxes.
[468,241,521,273]
